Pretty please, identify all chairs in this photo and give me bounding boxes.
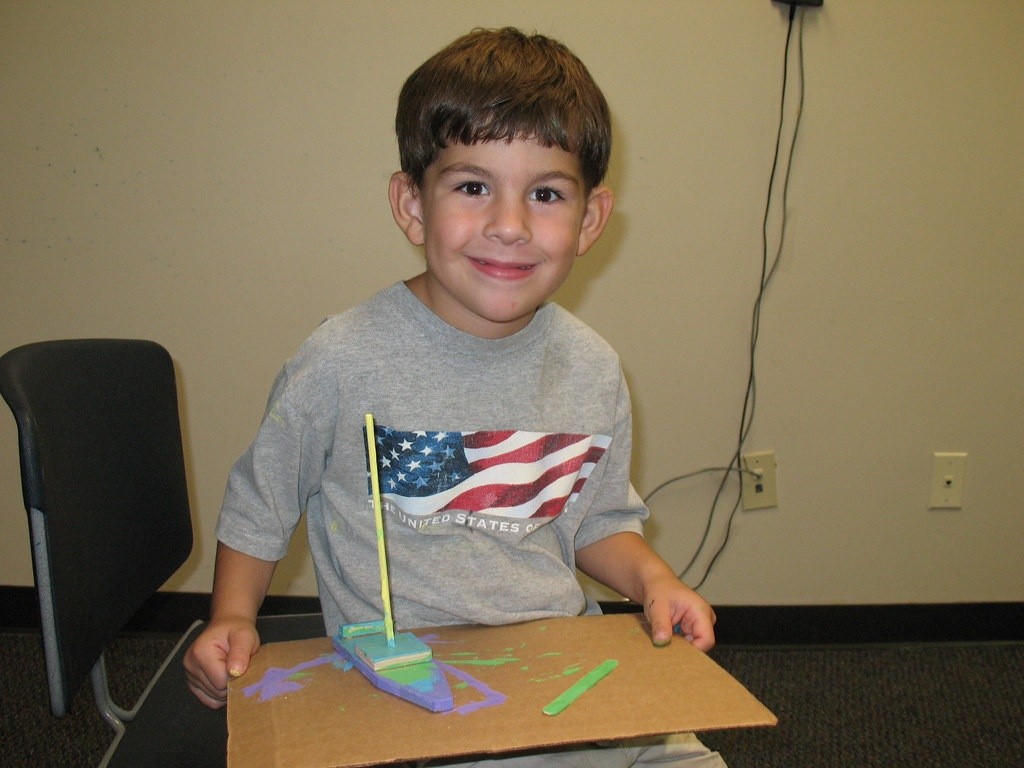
[0,338,324,768]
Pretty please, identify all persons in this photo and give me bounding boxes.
[182,24,730,768]
